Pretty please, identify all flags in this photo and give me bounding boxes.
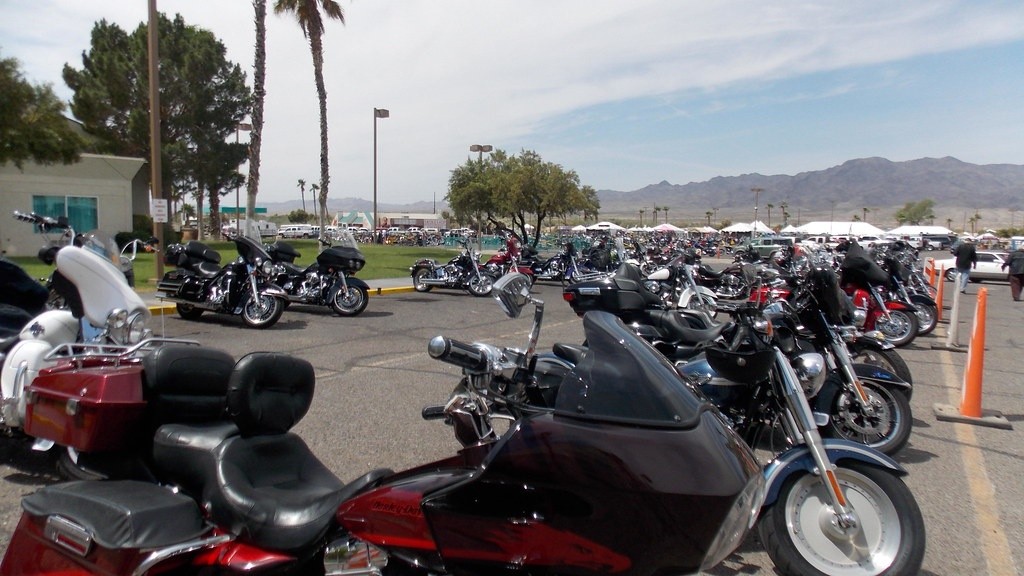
[375,109,389,118]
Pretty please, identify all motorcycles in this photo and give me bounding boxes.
[278,235,370,316]
[0,209,153,480]
[153,231,291,326]
[1,238,939,576]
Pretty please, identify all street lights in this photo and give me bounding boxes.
[373,109,390,244]
[470,144,493,250]
[751,188,766,238]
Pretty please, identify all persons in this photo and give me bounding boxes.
[1001,244,1024,300]
[950,238,977,294]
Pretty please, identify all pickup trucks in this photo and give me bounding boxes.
[800,236,840,252]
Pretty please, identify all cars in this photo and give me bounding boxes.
[924,239,942,252]
[862,235,895,250]
[278,225,477,238]
[932,251,1011,283]
[840,236,860,245]
[887,237,923,252]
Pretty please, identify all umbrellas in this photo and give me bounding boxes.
[524,223,534,230]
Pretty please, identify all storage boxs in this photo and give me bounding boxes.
[562,278,639,317]
[163,244,185,266]
[265,240,298,263]
[24,359,145,455]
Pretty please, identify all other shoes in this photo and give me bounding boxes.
[960,290,966,294]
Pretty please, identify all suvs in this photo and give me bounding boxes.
[734,237,793,261]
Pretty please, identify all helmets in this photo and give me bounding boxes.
[704,323,776,383]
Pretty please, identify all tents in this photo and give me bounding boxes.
[797,221,884,238]
[572,225,585,232]
[627,221,776,236]
[586,222,625,231]
[888,225,958,237]
[781,224,798,236]
